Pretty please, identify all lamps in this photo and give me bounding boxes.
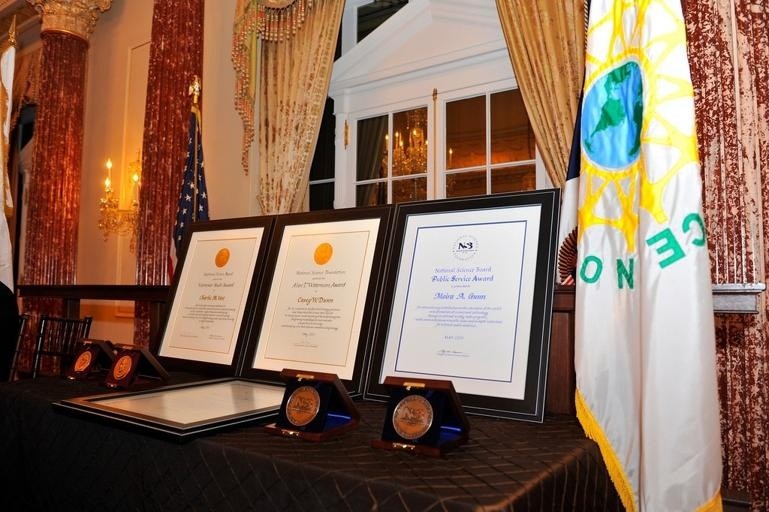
[381,111,456,201]
[94,159,140,256]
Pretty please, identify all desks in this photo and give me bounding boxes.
[0,371,626,512]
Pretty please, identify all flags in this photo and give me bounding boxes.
[555,0,724,512]
[166,106,209,286]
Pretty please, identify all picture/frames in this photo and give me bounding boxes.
[52,377,364,443]
[236,202,394,397]
[136,212,278,382]
[361,187,561,424]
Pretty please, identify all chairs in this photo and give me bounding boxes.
[31,314,92,380]
[8,316,27,384]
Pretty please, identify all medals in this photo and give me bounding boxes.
[75,350,92,372]
[392,395,433,439]
[286,385,320,426]
[113,355,132,380]
